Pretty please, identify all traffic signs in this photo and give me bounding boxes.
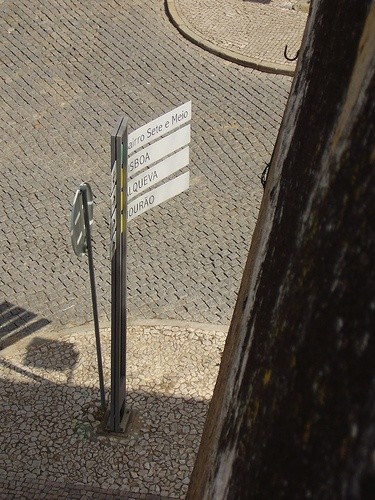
[127,98,191,155]
[69,182,94,257]
[127,147,189,201]
[110,232,117,261]
[109,162,117,195]
[127,171,189,224]
[110,210,117,240]
[109,186,117,215]
[126,124,190,178]
